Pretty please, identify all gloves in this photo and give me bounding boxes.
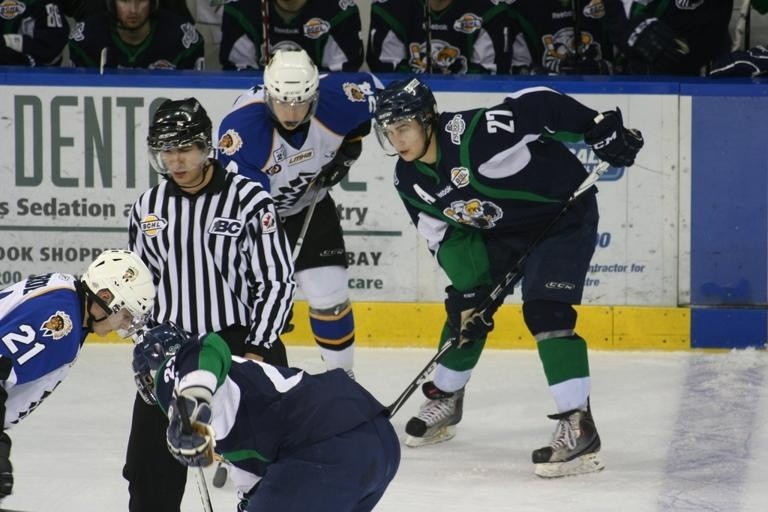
[585,106,644,167]
[444,283,495,350]
[0,430,14,501]
[313,136,362,190]
[165,396,216,468]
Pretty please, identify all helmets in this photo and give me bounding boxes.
[132,319,198,407]
[373,77,439,151]
[146,97,212,174]
[263,49,321,126]
[78,250,157,340]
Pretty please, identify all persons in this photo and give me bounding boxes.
[122,94,298,512]
[131,318,402,511]
[218,48,379,379]
[0,248,157,512]
[1,0,764,86]
[371,76,644,481]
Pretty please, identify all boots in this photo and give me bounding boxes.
[405,380,465,448]
[531,393,606,479]
